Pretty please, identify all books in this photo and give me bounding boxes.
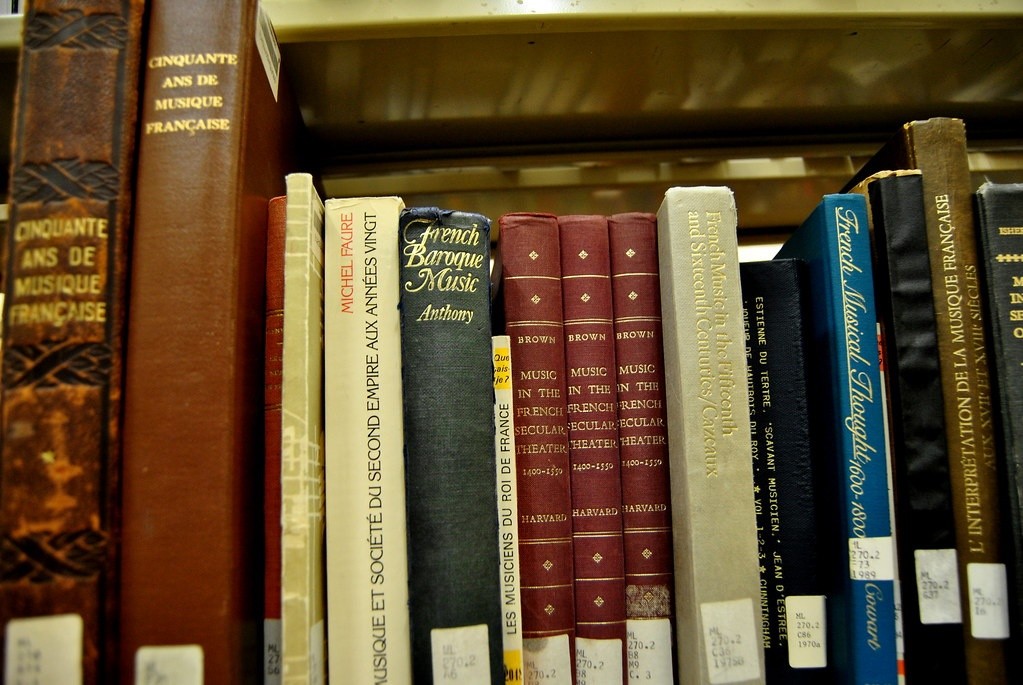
[0,0,1023,685]
